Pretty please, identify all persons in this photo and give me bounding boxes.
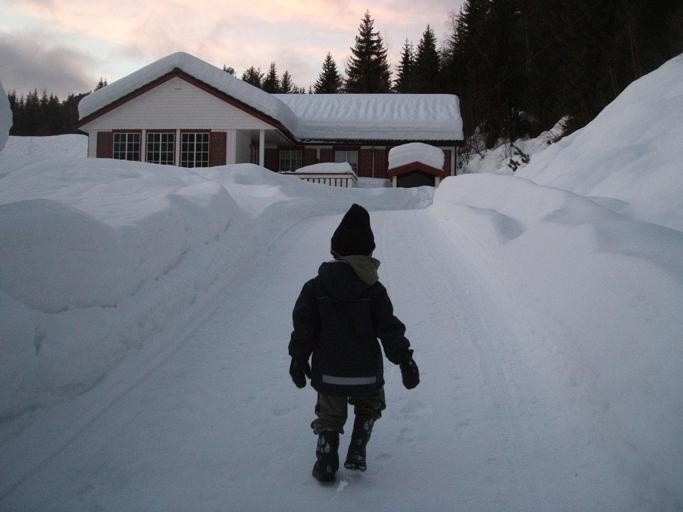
[287,204,419,483]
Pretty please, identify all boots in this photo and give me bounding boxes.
[344,415,378,471]
[312,431,344,483]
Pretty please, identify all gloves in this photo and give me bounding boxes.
[289,356,312,389]
[399,359,420,390]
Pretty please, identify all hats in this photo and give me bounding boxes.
[330,203,376,260]
